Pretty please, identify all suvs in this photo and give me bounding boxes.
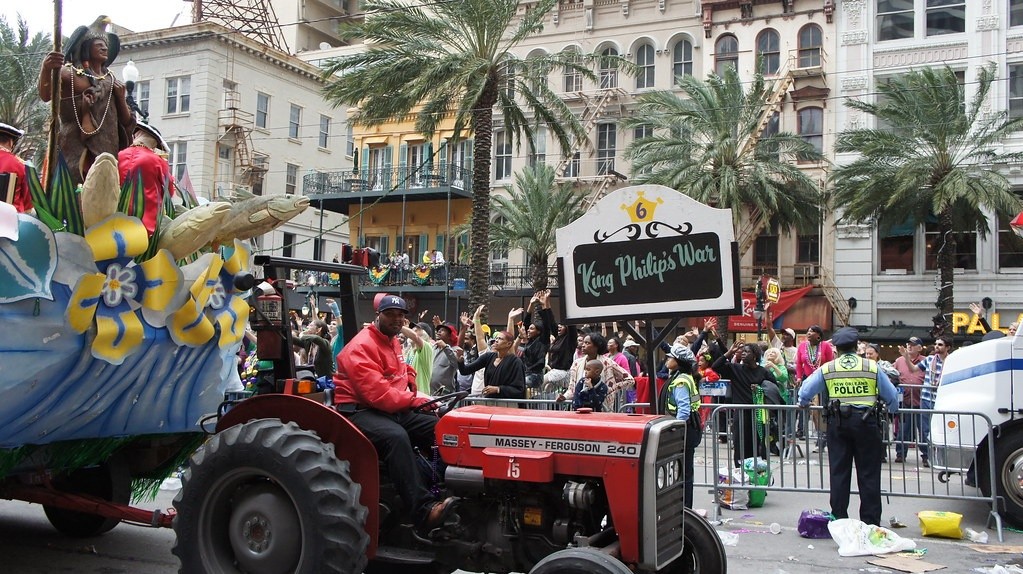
[927,317,1023,532]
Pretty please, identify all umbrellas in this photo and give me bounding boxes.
[352,146,359,179]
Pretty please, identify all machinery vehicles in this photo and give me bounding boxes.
[0,254,728,573]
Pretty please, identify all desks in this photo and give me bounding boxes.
[345,179,366,192]
[419,174,443,188]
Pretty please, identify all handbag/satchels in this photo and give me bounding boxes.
[743,458,775,508]
[827,519,916,556]
[917,510,964,541]
[717,469,750,510]
[798,510,835,539]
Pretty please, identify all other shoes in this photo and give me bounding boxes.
[895,455,905,464]
[429,497,455,524]
[923,459,929,467]
[881,456,886,462]
[811,446,825,453]
[795,430,803,438]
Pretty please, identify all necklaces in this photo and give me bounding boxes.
[405,348,415,365]
[127,142,153,150]
[70,65,114,134]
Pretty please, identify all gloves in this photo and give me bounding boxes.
[409,396,436,413]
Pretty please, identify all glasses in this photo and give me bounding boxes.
[933,342,947,346]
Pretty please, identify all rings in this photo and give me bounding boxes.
[484,391,487,394]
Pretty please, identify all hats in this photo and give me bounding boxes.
[577,327,590,335]
[666,344,698,367]
[778,327,795,340]
[906,336,923,348]
[831,327,860,347]
[133,123,171,155]
[408,321,432,338]
[0,120,21,139]
[378,295,408,315]
[437,322,458,338]
[623,340,640,349]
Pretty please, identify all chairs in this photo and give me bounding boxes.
[326,176,342,193]
[362,175,377,191]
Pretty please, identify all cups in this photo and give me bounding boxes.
[770,522,781,534]
[962,527,980,541]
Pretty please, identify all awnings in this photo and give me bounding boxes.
[364,129,468,145]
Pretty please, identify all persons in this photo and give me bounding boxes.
[365,287,646,413]
[332,253,339,263]
[118,121,176,236]
[384,249,410,282]
[432,249,445,262]
[38,14,136,220]
[239,293,344,392]
[0,122,34,215]
[856,302,1019,469]
[423,249,432,263]
[333,295,472,528]
[797,326,899,528]
[653,314,835,512]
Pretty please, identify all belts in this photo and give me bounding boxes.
[839,406,866,414]
